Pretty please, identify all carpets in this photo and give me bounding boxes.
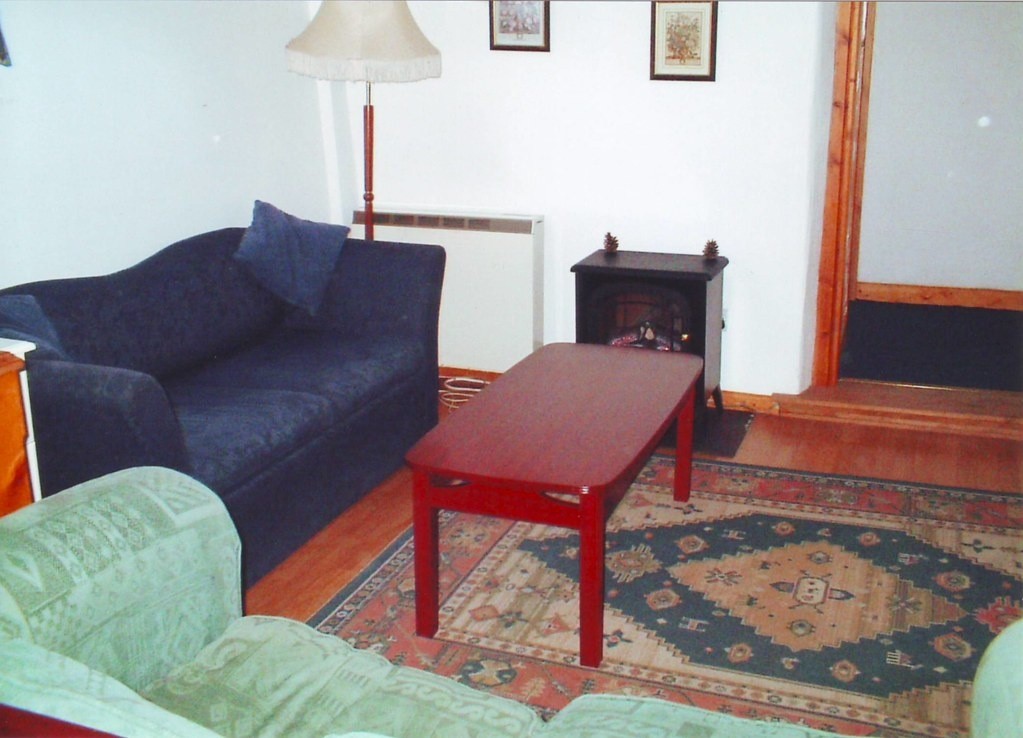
[305,453,1023,738]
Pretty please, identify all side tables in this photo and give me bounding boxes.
[0,335,43,519]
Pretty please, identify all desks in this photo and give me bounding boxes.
[405,344,704,668]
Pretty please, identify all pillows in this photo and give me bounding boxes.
[235,200,350,315]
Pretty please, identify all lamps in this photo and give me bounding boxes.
[282,0,442,240]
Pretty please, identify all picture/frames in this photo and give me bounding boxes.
[489,0,550,52]
[649,0,718,82]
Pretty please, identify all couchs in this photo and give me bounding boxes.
[0,200,447,594]
[0,466,1023,738]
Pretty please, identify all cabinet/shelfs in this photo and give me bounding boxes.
[569,250,728,434]
